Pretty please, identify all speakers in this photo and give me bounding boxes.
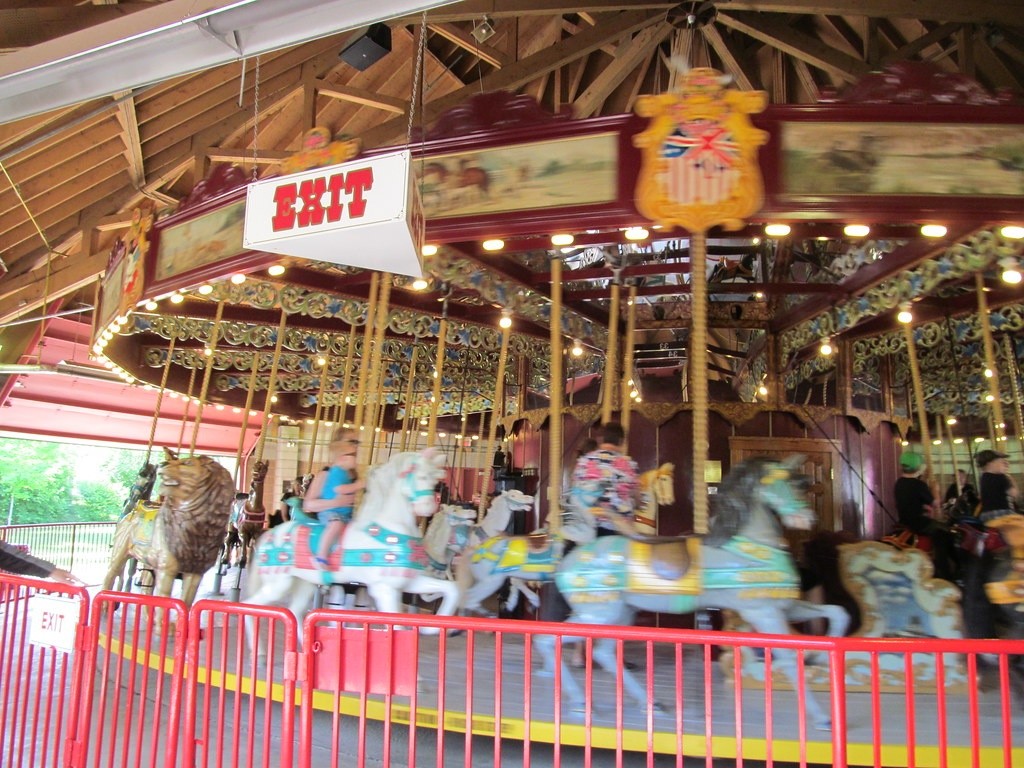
[340,23,393,71]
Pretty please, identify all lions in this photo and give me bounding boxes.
[100,452,237,638]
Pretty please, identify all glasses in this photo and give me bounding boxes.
[340,439,359,445]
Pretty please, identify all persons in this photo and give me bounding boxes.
[281,428,365,584]
[978,450,1018,522]
[563,419,642,558]
[945,470,981,519]
[894,452,958,587]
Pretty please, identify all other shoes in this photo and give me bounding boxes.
[624,660,637,672]
[309,556,330,572]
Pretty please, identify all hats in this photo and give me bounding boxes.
[976,449,1010,465]
[899,451,924,470]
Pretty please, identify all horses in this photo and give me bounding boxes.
[231,453,850,638]
